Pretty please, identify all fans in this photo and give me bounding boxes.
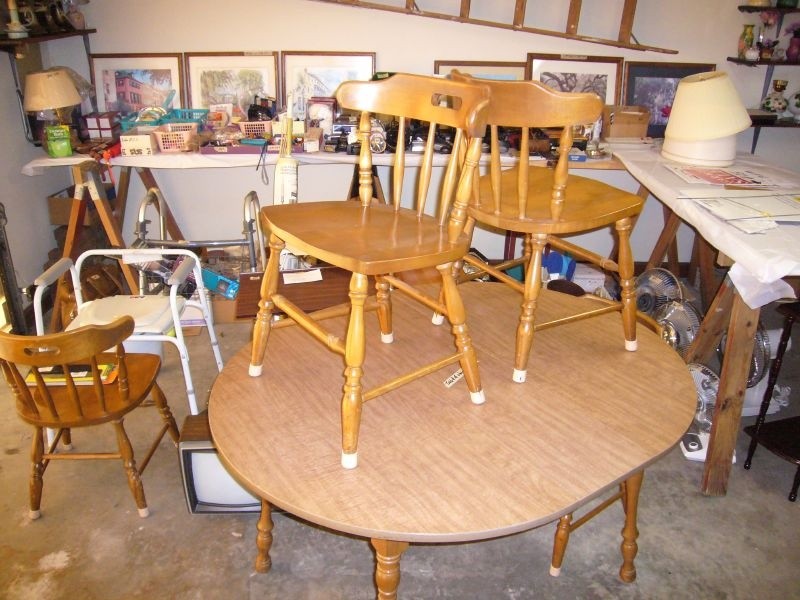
[633,268,792,464]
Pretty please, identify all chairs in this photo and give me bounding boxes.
[246,70,491,470]
[431,68,644,384]
[0,315,180,521]
[33,248,226,416]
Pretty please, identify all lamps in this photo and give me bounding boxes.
[660,69,752,168]
[22,69,84,152]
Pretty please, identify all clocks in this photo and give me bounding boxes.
[305,97,337,136]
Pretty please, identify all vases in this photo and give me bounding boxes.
[737,25,755,60]
[785,38,800,63]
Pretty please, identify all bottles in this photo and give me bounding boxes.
[738,25,755,59]
[788,91,800,120]
[66,5,85,30]
[761,92,789,113]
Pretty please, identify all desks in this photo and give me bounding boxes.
[599,136,800,498]
[205,283,697,600]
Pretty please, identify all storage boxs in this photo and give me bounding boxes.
[46,183,118,227]
[271,115,304,137]
[332,115,384,134]
[120,125,161,157]
[603,106,649,137]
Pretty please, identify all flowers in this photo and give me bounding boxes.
[757,8,781,48]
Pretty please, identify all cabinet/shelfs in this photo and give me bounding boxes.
[742,300,800,502]
[727,0,800,155]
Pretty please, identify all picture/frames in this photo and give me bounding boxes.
[86,51,718,143]
